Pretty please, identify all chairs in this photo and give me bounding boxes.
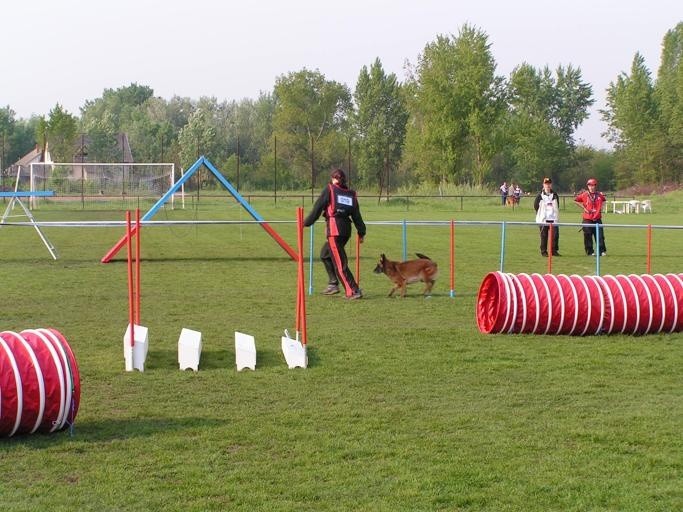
[629,200,652,214]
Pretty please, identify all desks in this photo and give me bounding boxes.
[602,201,608,213]
[611,201,629,214]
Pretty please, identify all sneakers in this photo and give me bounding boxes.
[542,252,562,257]
[343,289,363,300]
[591,251,607,256]
[320,285,340,295]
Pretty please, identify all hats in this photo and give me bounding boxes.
[543,177,552,183]
[586,178,598,186]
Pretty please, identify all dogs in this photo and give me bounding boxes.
[373,253,438,298]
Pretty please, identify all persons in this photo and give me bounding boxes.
[572,178,607,257]
[533,178,561,257]
[302,168,366,301]
[499,182,523,207]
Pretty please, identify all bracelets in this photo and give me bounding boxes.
[583,207,584,210]
